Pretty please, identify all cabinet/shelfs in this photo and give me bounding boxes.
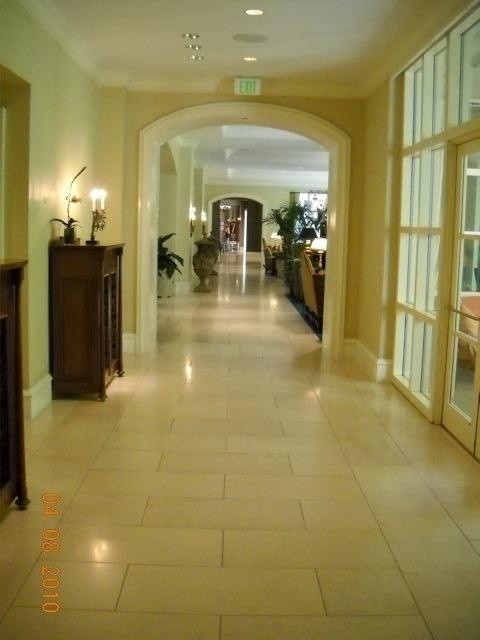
[47,238,126,402]
[1,257,31,524]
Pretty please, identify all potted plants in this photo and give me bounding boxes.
[48,165,88,244]
[158,231,185,299]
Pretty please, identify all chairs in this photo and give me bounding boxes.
[261,236,327,331]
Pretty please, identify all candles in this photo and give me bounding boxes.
[90,186,99,213]
[99,187,107,212]
